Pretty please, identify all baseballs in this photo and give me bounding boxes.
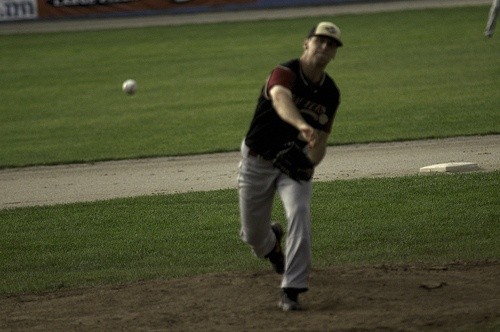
[122,79,137,95]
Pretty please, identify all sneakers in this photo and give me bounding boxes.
[267,223,288,273]
[276,286,304,310]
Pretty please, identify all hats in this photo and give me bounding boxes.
[306,20,344,48]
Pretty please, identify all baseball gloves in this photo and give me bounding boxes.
[272,142,315,186]
